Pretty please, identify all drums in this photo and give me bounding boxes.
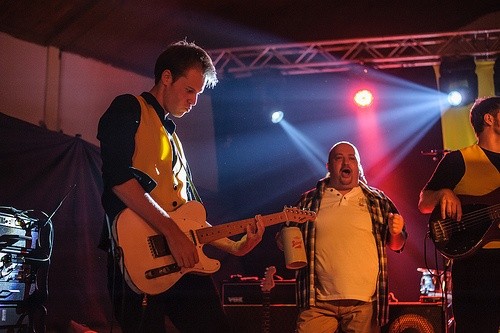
[420,270,453,299]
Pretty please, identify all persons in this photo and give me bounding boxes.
[273,140,409,333]
[417,95,500,333]
[96,40,266,333]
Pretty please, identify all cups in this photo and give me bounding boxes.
[281,227,308,269]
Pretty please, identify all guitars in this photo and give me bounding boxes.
[110,205,317,297]
[260,265,276,333]
[428,186,500,259]
